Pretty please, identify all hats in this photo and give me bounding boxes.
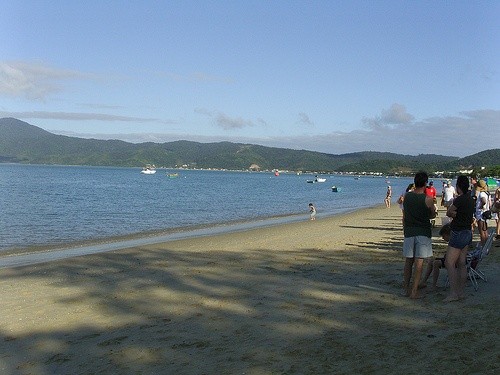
[447,181,451,184]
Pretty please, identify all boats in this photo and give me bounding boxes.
[166,173,179,178]
[353,177,360,180]
[141,168,158,174]
[306,180,315,183]
[384,179,391,183]
[315,178,327,182]
[332,186,342,192]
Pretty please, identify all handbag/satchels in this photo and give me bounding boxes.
[397,196,404,204]
[482,210,492,219]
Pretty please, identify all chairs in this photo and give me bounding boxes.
[444,228,496,292]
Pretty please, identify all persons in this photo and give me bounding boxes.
[397,175,500,248]
[309,203,316,220]
[402,173,436,299]
[411,223,468,290]
[442,176,474,302]
[384,186,392,208]
